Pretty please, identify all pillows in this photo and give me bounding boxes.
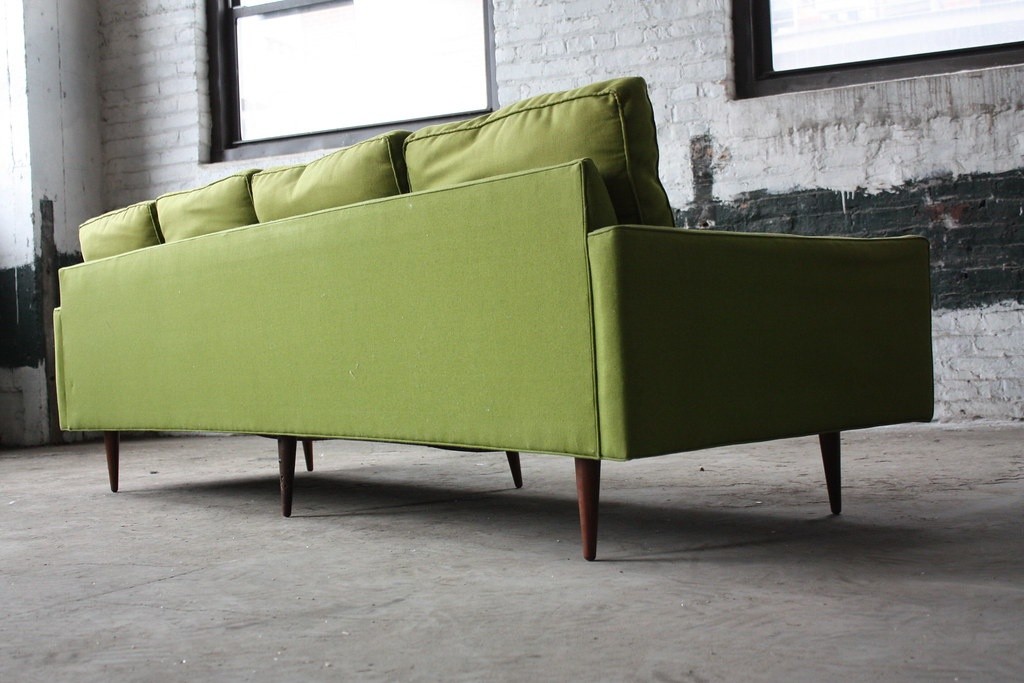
[252,129,413,224]
[78,199,165,263]
[154,166,262,246]
[401,77,674,228]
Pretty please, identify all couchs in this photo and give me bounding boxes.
[51,154,938,561]
